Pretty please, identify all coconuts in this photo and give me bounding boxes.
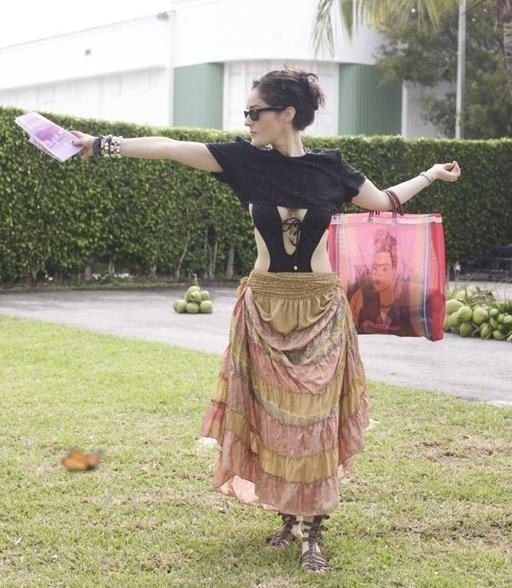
[173,285,214,314]
[443,283,512,342]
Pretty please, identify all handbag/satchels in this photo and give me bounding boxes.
[328,210,447,340]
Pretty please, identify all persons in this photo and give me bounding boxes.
[69,70,461,573]
[350,236,411,337]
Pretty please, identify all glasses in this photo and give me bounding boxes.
[244,106,283,119]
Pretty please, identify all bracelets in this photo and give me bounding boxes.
[420,172,434,185]
[92,135,124,160]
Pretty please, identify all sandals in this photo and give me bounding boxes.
[299,514,330,572]
[267,513,299,551]
